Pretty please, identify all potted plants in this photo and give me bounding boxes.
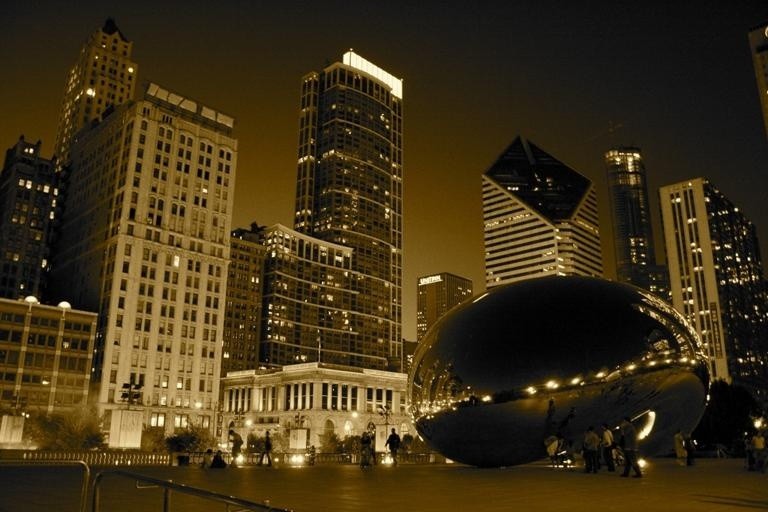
[163,432,201,467]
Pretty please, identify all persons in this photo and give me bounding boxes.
[672,429,696,466]
[256,431,272,467]
[366,436,377,465]
[229,430,243,468]
[544,417,643,478]
[201,449,213,469]
[546,399,576,433]
[385,428,400,465]
[212,450,226,468]
[744,431,768,473]
[360,432,371,466]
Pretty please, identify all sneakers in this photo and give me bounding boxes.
[620,474,629,477]
[632,474,642,478]
